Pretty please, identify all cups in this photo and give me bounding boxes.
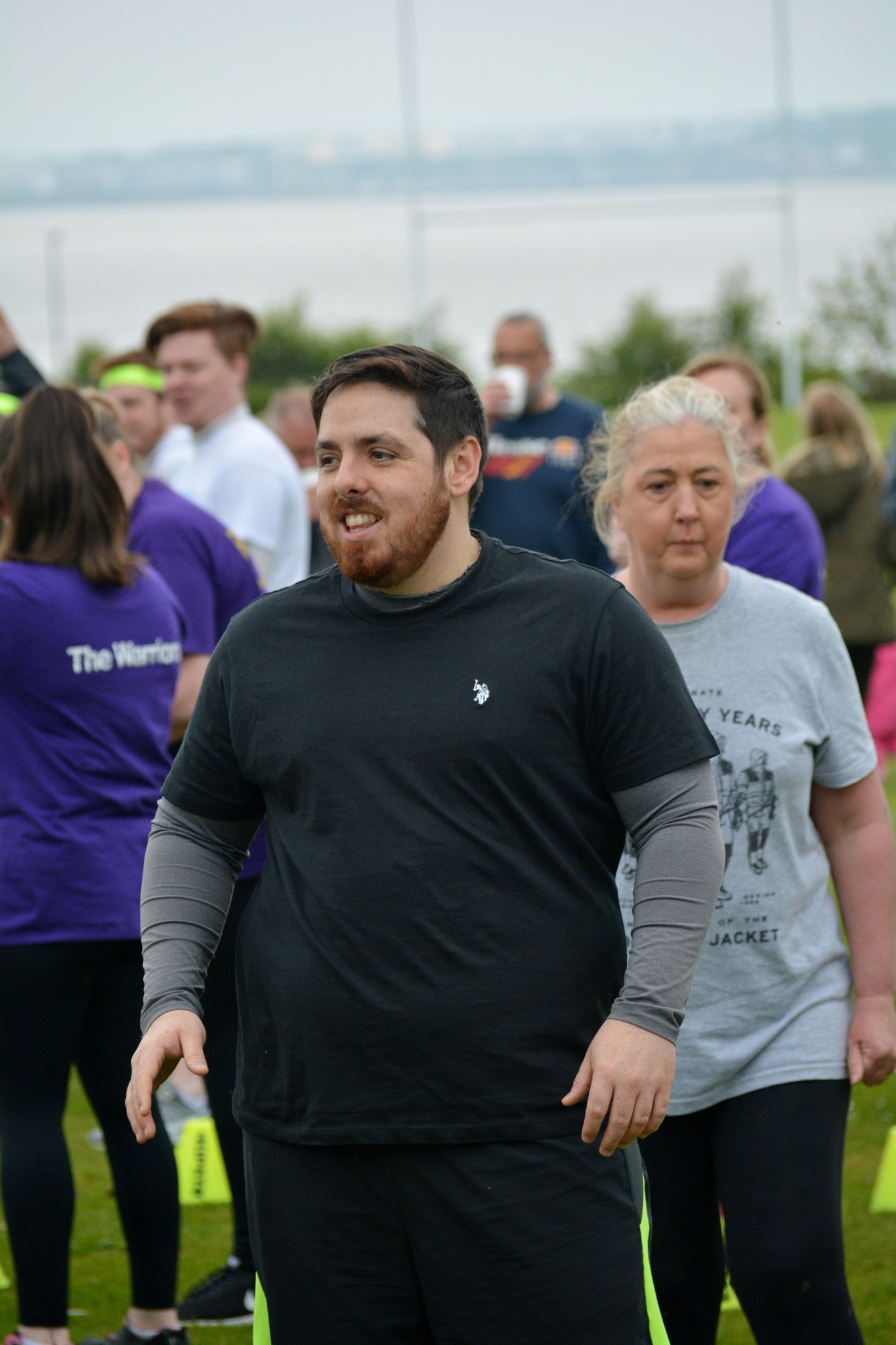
[494,364,529,418]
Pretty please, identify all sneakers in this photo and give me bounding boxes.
[176,1257,256,1326]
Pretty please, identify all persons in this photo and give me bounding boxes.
[125,343,725,1345]
[473,311,615,575]
[0,389,184,1345]
[139,304,311,594]
[258,383,315,522]
[780,380,896,772]
[589,371,896,1345]
[93,355,188,474]
[0,304,269,1326]
[678,353,828,597]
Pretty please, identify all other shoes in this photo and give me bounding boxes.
[93,1082,213,1148]
[80,1324,189,1345]
